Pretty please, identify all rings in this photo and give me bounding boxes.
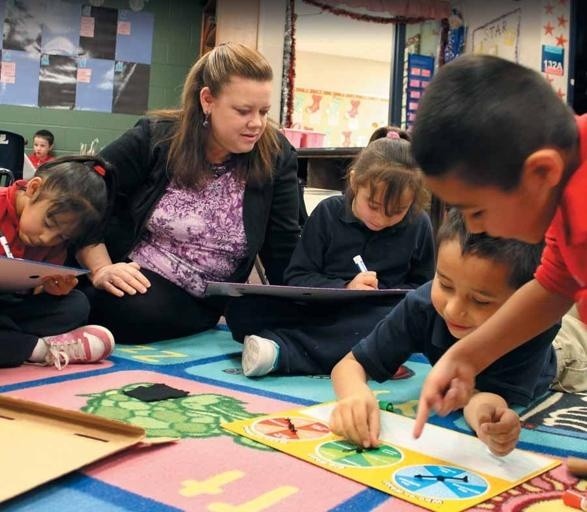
[109,274,115,283]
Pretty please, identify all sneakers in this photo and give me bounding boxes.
[241,335,279,377]
[24,324,116,370]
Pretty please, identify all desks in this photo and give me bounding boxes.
[293,147,365,187]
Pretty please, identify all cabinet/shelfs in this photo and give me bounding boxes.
[400,50,435,134]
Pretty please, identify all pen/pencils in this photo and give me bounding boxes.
[0,236,14,258]
[352,254,368,272]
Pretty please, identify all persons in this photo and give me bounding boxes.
[331,208,587,456]
[409,53,587,438]
[0,155,119,369]
[225,127,437,377]
[28,130,57,170]
[65,41,303,345]
